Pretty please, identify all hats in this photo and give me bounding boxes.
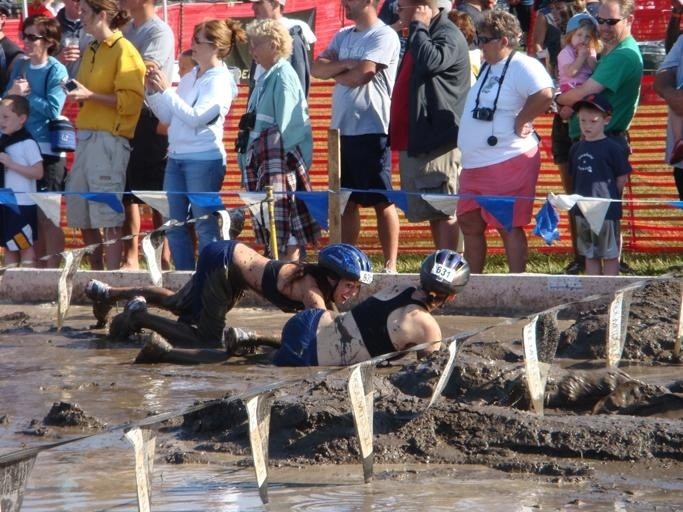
[242,0,286,5]
[570,93,613,115]
[566,13,597,33]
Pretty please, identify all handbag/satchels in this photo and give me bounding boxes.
[46,116,77,152]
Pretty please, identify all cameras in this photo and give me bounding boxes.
[472,106,495,121]
[235,130,249,154]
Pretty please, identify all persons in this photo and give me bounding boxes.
[310,1,682,273]
[86,240,373,346]
[134,247,474,365]
[2,2,318,272]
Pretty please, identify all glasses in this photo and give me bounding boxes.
[191,38,215,45]
[596,16,624,25]
[19,33,45,41]
[474,37,498,45]
[395,4,418,12]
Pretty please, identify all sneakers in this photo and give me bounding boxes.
[134,331,172,364]
[224,327,251,355]
[563,256,585,274]
[110,296,146,343]
[619,262,629,273]
[85,278,112,322]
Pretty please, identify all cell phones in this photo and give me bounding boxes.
[65,81,76,90]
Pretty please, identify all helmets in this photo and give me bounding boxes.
[319,243,373,284]
[419,249,470,296]
